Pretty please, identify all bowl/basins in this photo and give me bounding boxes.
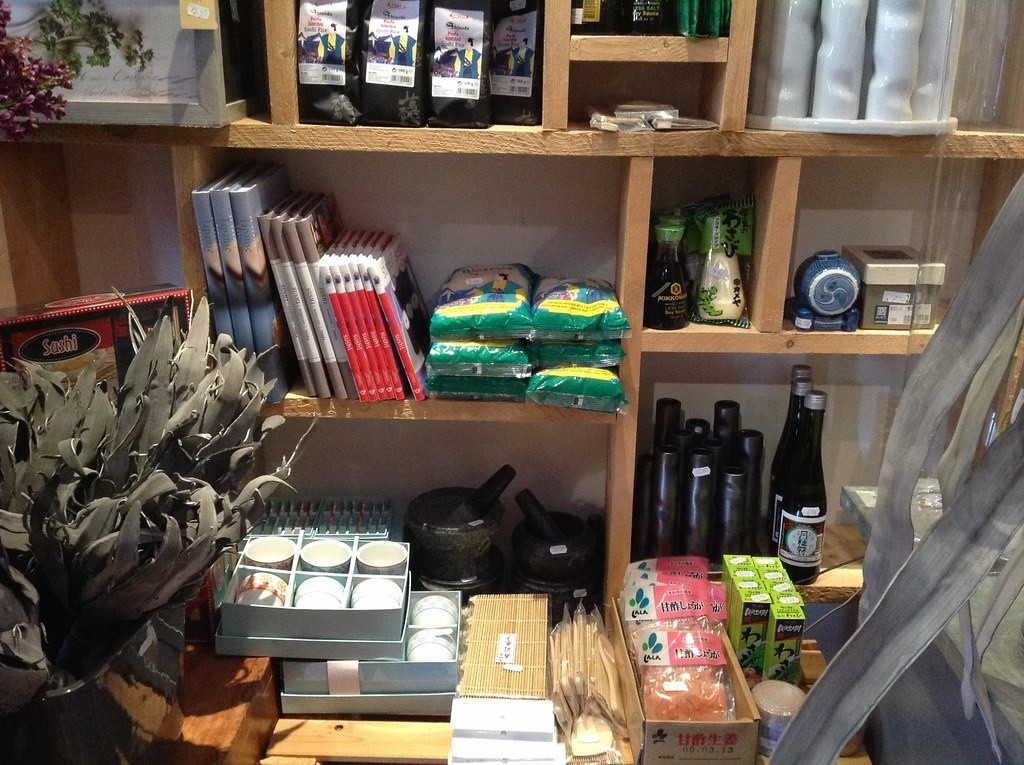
[357,541,408,575]
[350,578,402,608]
[294,577,345,607]
[411,595,457,625]
[300,540,352,574]
[235,572,287,607]
[406,629,456,662]
[244,537,296,571]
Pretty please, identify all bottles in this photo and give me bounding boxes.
[770,377,813,556]
[643,214,689,330]
[778,390,827,585]
[767,364,811,533]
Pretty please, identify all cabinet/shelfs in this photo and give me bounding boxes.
[0,0,1024,765]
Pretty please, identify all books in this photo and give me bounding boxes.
[191,159,428,403]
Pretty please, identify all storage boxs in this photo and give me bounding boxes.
[0,283,189,395]
[840,243,946,329]
[608,597,762,765]
[722,555,806,683]
[213,496,462,717]
